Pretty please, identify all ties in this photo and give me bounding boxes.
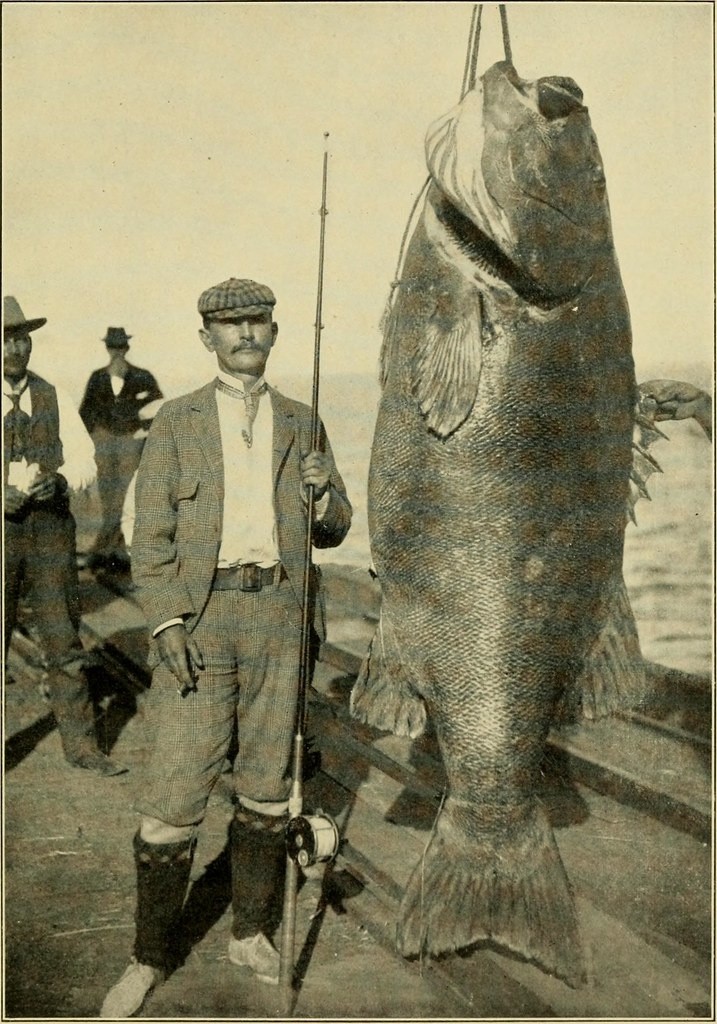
[216,379,267,448]
[3,382,31,484]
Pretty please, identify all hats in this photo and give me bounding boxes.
[4,296,47,332]
[198,277,276,318]
[102,327,132,341]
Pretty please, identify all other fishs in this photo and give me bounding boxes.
[347,52,669,993]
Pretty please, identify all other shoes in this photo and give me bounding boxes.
[228,930,280,985]
[70,748,127,777]
[100,955,164,1017]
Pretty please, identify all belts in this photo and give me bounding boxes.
[212,561,288,592]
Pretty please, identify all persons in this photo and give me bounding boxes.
[98,280,353,1018]
[77,328,165,577]
[1,294,127,778]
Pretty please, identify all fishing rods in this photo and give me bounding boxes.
[275,129,333,1015]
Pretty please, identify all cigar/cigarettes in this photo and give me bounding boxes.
[176,672,195,696]
